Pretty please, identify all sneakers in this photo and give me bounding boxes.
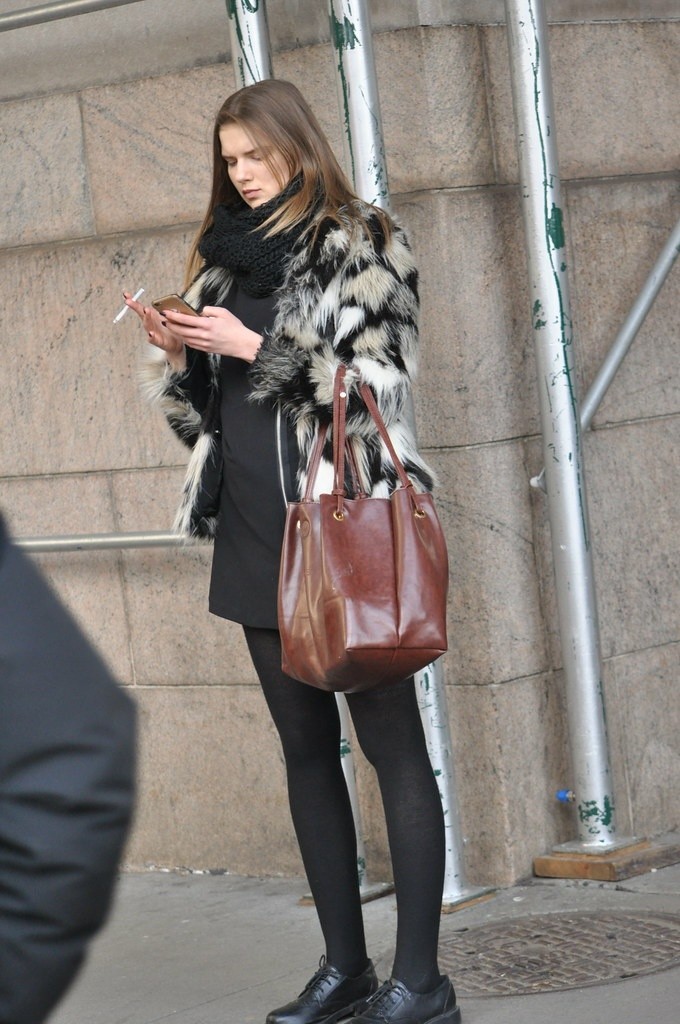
[266,954,377,1024]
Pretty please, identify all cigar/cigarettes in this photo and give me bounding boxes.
[113,289,145,324]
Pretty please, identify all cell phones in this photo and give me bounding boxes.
[152,293,202,318]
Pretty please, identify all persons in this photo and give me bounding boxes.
[0,512,138,1024]
[123,79,460,1024]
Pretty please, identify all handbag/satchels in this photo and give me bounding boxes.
[276,365,450,692]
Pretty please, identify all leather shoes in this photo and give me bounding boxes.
[344,974,462,1024]
